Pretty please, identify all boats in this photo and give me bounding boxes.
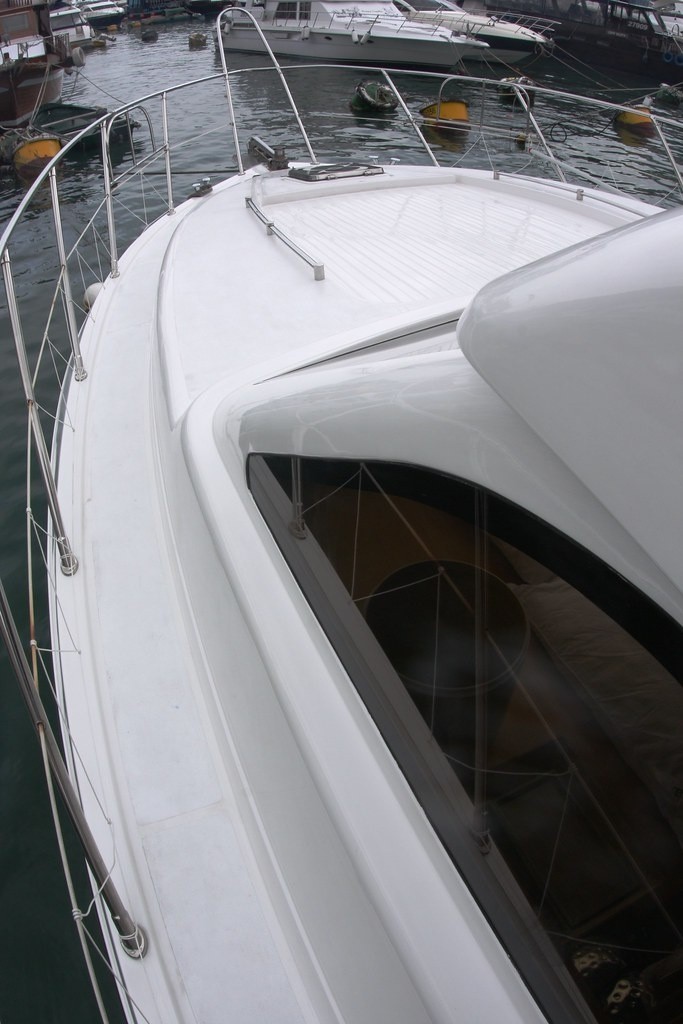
[49,0,92,48]
[211,0,491,74]
[73,0,125,28]
[29,101,143,155]
[0,0,87,134]
[392,0,562,66]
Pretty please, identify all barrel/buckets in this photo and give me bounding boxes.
[365,558,531,742]
